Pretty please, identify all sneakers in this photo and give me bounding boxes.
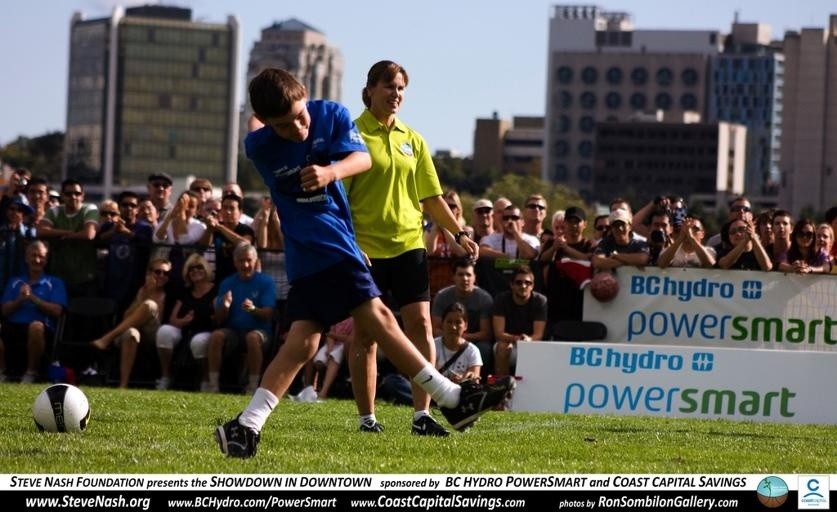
[360,420,383,434]
[440,374,519,432]
[215,412,262,458]
[21,373,35,384]
[153,372,259,396]
[410,413,450,436]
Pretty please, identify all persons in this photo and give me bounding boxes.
[341,58,482,437]
[0,166,836,410]
[217,65,517,456]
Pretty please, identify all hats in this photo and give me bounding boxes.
[609,208,630,225]
[148,170,173,186]
[564,206,587,221]
[472,198,493,211]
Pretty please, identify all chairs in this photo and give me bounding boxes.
[240,309,279,358]
[58,297,117,385]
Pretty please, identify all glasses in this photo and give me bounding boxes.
[596,224,608,231]
[730,226,746,234]
[502,214,520,222]
[526,203,546,211]
[796,230,813,239]
[514,279,533,286]
[188,264,205,272]
[151,268,170,276]
[730,205,752,212]
[65,182,212,217]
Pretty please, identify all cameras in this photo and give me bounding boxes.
[17,174,31,189]
[665,204,687,229]
[649,228,670,244]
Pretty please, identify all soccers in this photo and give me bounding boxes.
[32,385,91,432]
